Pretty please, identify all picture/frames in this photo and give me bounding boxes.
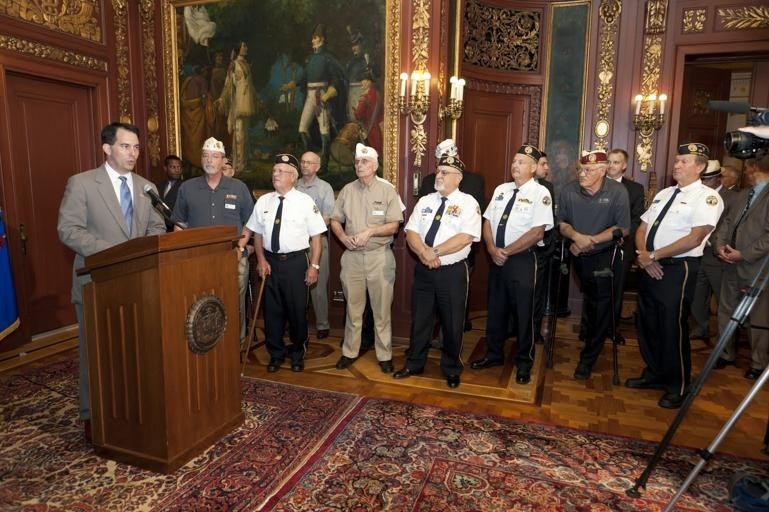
[160,0,401,196]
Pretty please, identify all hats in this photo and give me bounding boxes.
[312,22,327,36]
[273,153,299,171]
[202,136,226,156]
[437,152,465,174]
[579,149,610,165]
[701,159,726,179]
[675,142,710,160]
[434,138,458,159]
[516,142,542,164]
[355,142,379,160]
[225,154,233,168]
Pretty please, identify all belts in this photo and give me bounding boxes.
[263,247,311,262]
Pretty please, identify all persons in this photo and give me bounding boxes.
[188,25,384,185]
[56,122,167,446]
[391,137,768,411]
[172,136,408,372]
[154,156,188,209]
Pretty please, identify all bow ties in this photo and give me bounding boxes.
[271,196,284,253]
[119,176,134,238]
[164,181,172,198]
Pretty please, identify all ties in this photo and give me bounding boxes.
[424,196,448,248]
[728,188,755,250]
[645,189,681,252]
[496,188,520,248]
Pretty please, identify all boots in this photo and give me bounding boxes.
[300,132,313,153]
[316,133,332,177]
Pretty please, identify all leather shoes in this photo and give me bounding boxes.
[84,420,94,445]
[625,376,696,410]
[267,356,285,372]
[574,361,593,380]
[471,352,506,370]
[534,333,545,345]
[317,329,329,339]
[290,357,304,372]
[516,365,531,384]
[610,333,626,346]
[336,354,359,369]
[712,356,736,370]
[446,373,460,389]
[378,358,425,379]
[745,367,765,380]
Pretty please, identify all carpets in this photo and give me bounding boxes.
[0,343,769,512]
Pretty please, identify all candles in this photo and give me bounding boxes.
[396,70,465,100]
[633,92,666,114]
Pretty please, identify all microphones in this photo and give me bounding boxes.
[144,184,169,209]
